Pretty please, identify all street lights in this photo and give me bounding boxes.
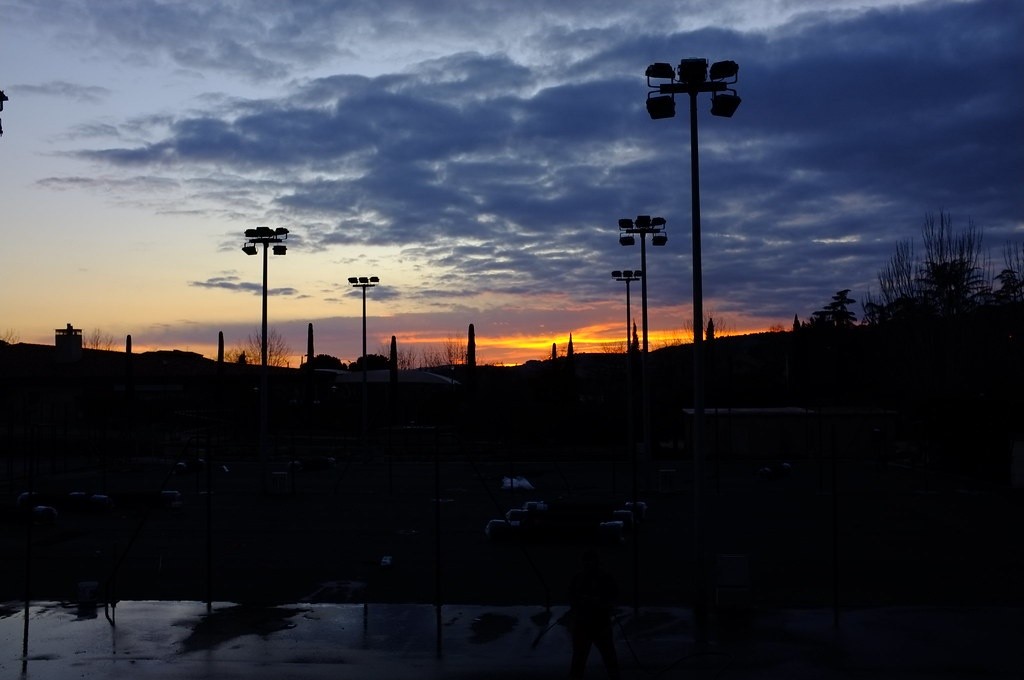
[350,275,380,380]
[610,268,641,352]
[642,54,742,419]
[244,225,288,367]
[620,212,667,352]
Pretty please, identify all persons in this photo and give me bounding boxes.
[561,548,625,680]
